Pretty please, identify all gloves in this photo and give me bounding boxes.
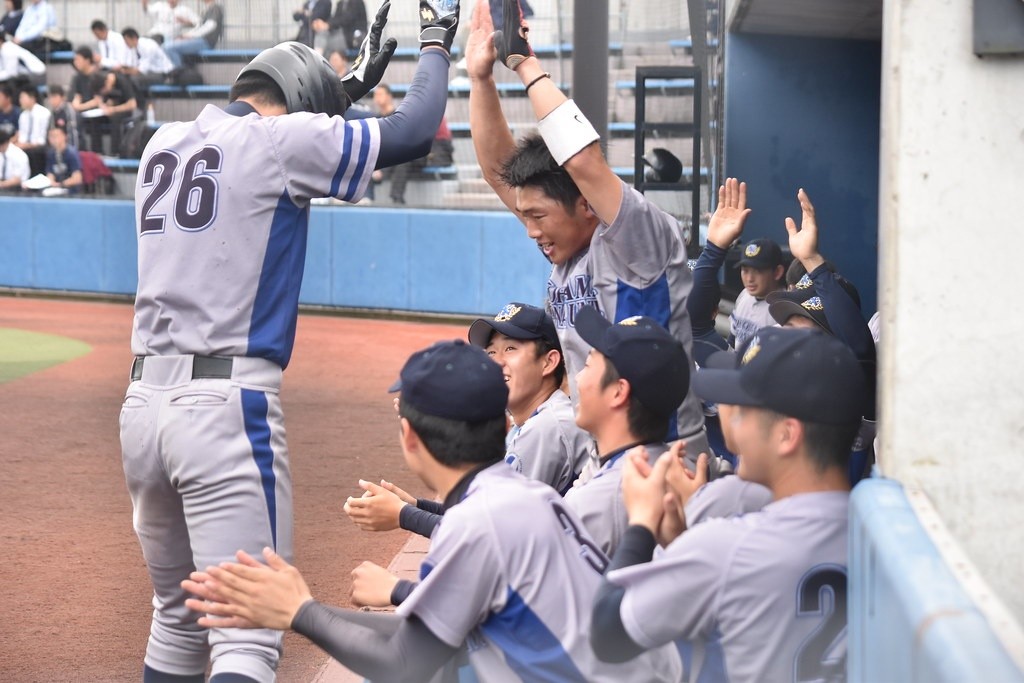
[341,0,398,106]
[488,0,535,72]
[418,0,460,55]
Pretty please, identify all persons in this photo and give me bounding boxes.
[27,128,84,198]
[179,337,684,683]
[685,177,875,489]
[466,0,715,483]
[343,301,589,538]
[0,0,222,191]
[120,0,510,683]
[349,303,690,606]
[631,327,792,683]
[589,330,866,683]
[0,130,31,189]
[293,0,454,206]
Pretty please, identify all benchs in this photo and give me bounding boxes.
[36,37,723,213]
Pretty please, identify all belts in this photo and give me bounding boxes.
[131,357,233,381]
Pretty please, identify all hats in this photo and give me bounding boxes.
[786,256,835,292]
[386,340,510,424]
[573,303,691,412]
[736,239,784,269]
[768,295,835,339]
[687,326,867,426]
[468,302,560,353]
[704,322,777,372]
[766,273,861,316]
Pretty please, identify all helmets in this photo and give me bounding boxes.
[234,41,348,118]
[642,147,683,185]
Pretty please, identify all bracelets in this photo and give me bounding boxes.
[525,72,551,93]
[61,181,63,188]
